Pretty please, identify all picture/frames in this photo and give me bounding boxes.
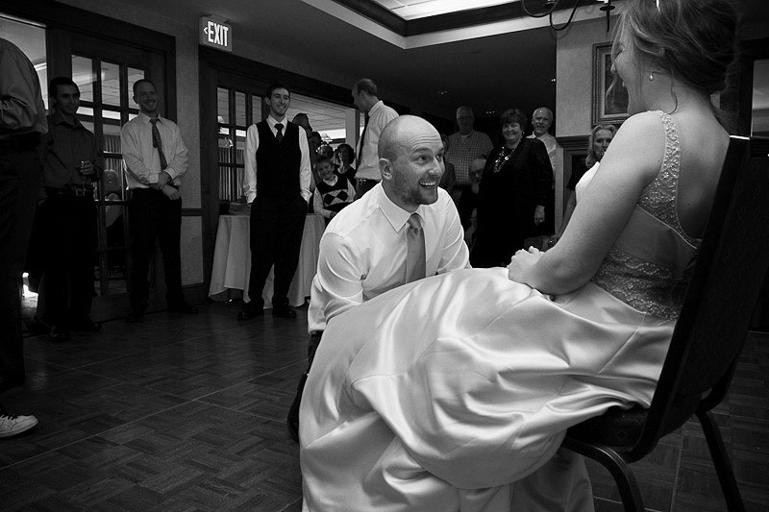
[591,40,720,125]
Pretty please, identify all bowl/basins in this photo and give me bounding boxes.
[228,201,248,215]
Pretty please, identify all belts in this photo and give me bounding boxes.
[44,187,92,198]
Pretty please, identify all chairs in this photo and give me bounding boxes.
[541,136,768,512]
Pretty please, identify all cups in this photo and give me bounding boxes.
[80,160,89,174]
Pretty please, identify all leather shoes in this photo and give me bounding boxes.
[167,303,198,315]
[236,305,264,321]
[273,305,298,318]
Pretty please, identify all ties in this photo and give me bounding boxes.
[352,111,369,175]
[405,212,425,284]
[149,117,168,171]
[274,123,285,143]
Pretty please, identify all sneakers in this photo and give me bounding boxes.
[0,413,39,438]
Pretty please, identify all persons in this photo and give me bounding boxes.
[292,79,400,228]
[440,106,617,268]
[38,76,105,339]
[237,81,312,321]
[299,1,731,512]
[0,39,45,393]
[287,115,472,446]
[121,79,200,323]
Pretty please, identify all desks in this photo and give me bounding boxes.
[208,214,326,310]
